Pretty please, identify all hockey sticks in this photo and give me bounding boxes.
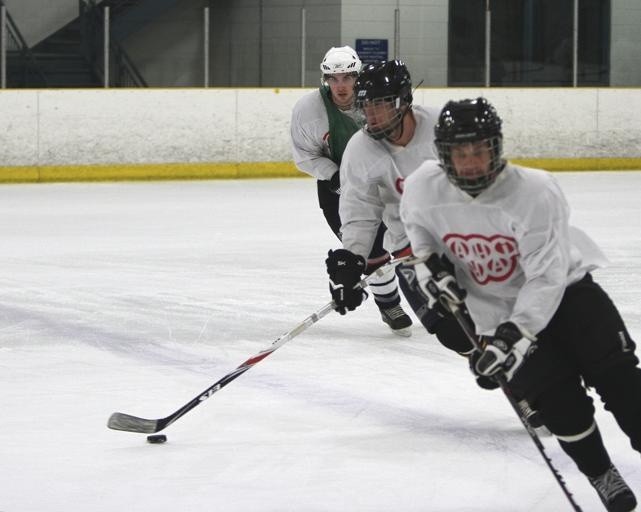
[108,255,414,432]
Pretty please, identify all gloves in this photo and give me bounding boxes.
[414,253,540,391]
[325,248,367,316]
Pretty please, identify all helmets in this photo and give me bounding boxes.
[320,46,414,142]
[434,96,505,196]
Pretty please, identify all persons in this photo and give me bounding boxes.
[288,43,434,331]
[323,57,443,332]
[399,97,640,512]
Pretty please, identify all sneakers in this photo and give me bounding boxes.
[508,393,554,438]
[379,304,414,337]
[588,461,640,512]
